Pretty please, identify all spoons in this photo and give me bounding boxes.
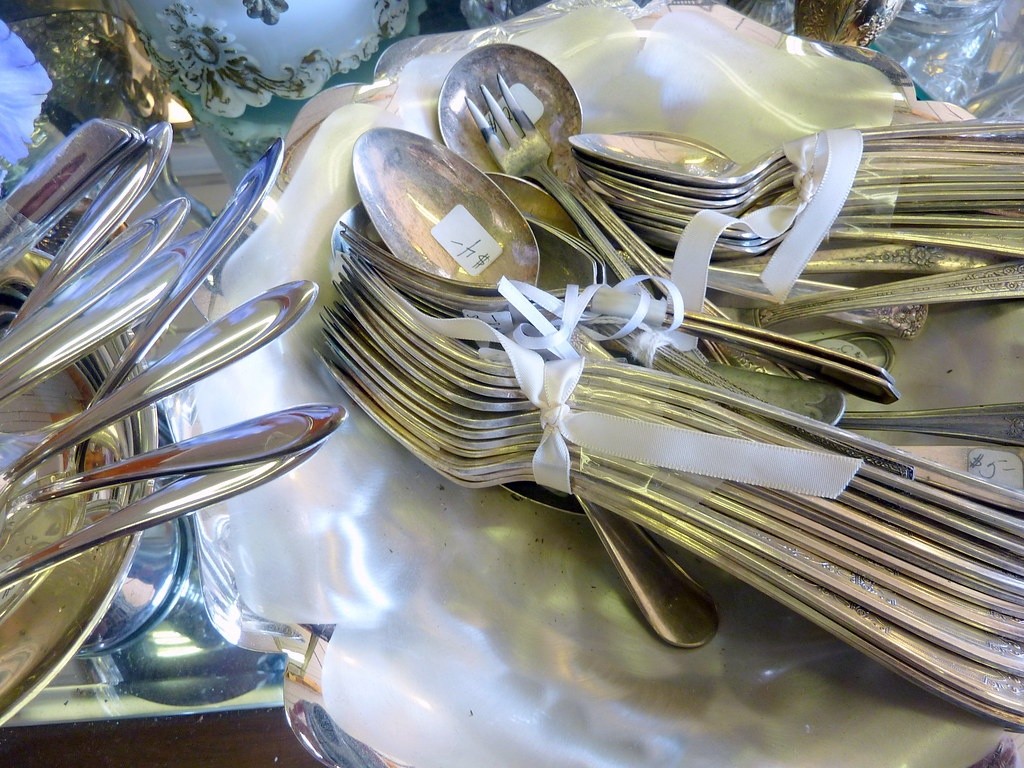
[0,44,1024,732]
